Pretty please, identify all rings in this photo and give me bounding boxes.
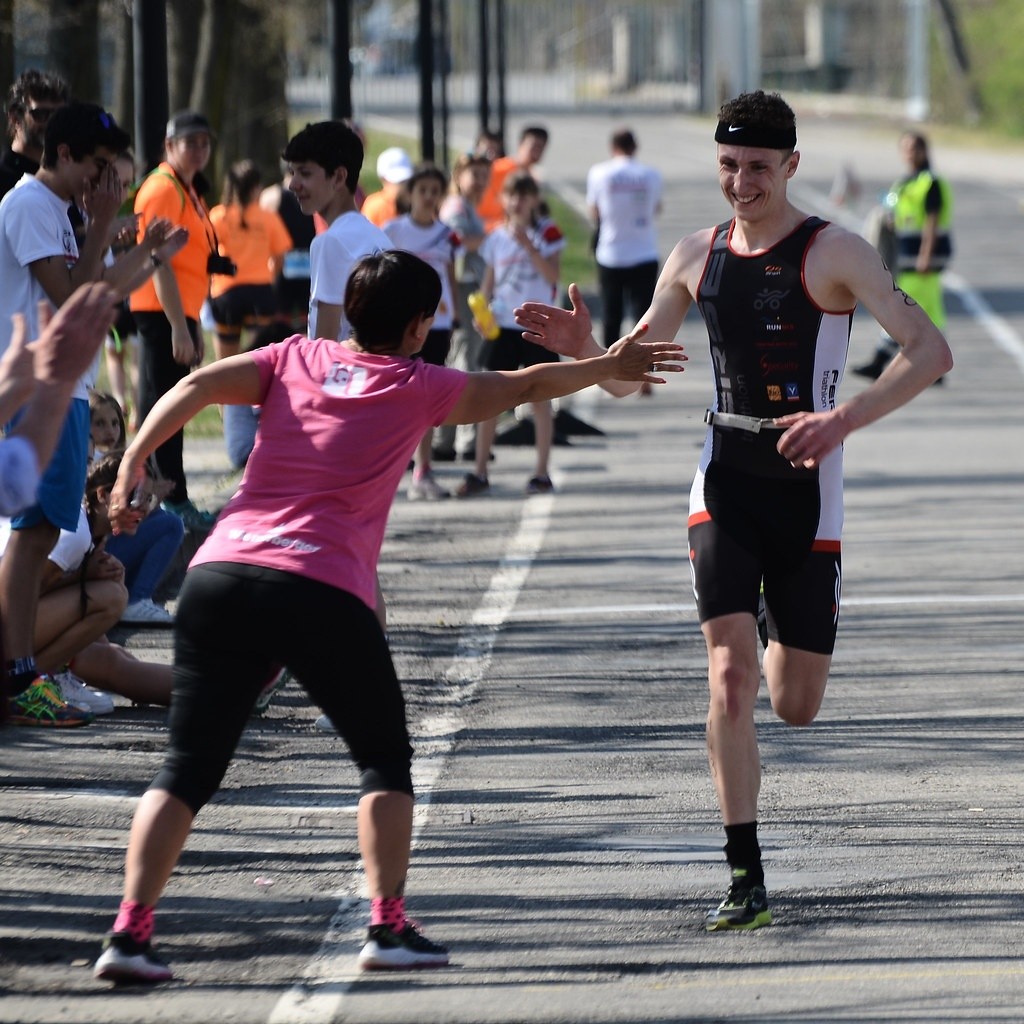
[652,364,658,372]
[111,505,120,510]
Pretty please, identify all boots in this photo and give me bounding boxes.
[933,377,945,385]
[850,349,893,381]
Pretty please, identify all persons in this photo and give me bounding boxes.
[845,130,954,392]
[0,66,566,732]
[586,129,667,396]
[510,88,958,938]
[91,244,691,981]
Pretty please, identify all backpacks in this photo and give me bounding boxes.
[110,169,186,264]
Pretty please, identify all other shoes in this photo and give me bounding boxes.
[463,451,495,461]
[431,449,456,460]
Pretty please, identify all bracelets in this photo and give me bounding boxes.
[149,249,162,272]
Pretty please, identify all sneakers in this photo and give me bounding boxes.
[5,677,95,728]
[121,599,173,623]
[706,868,771,932]
[51,670,114,715]
[160,501,215,532]
[454,474,491,499]
[409,479,450,502]
[358,919,450,971]
[527,474,553,492]
[92,930,173,981]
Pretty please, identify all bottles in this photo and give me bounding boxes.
[467,293,500,340]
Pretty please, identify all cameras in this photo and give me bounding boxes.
[206,252,237,276]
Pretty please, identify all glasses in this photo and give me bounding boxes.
[26,107,50,122]
[99,111,116,130]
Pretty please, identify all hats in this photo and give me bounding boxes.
[376,147,413,184]
[167,113,213,138]
